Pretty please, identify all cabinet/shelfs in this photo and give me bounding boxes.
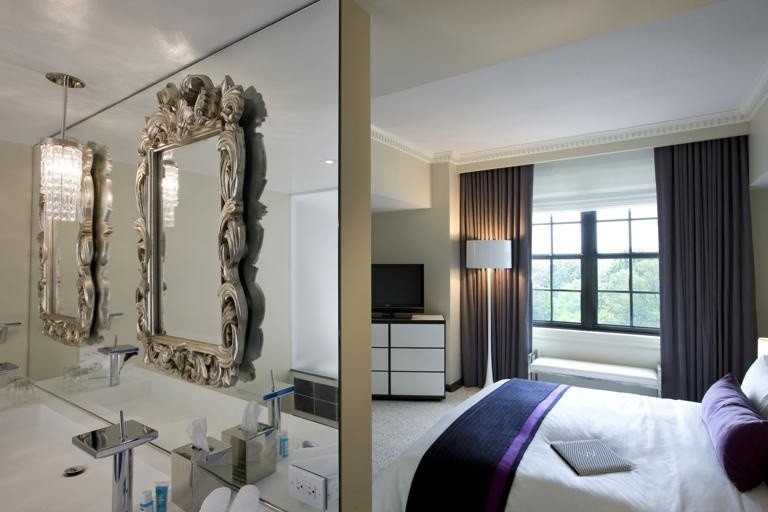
[370,313,447,402]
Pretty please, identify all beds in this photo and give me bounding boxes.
[370,337,768,512]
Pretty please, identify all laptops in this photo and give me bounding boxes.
[549,438,633,476]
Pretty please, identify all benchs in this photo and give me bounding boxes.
[527,349,663,398]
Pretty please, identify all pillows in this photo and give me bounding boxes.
[701,373,767,492]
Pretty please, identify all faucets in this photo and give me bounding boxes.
[97,333,140,387]
[259,369,297,433]
[69,411,158,512]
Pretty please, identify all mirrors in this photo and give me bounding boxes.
[34,137,95,347]
[133,72,249,389]
[29,0,340,511]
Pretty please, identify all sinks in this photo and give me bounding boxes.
[75,375,267,452]
[1,405,177,511]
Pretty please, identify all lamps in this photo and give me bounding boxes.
[465,238,512,389]
[40,71,86,223]
[159,150,180,229]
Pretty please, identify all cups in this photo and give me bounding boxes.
[7,377,23,390]
[71,368,88,385]
[63,367,79,382]
[17,378,32,393]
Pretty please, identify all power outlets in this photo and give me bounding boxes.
[287,464,327,512]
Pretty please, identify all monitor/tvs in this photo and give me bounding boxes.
[372,262,425,319]
